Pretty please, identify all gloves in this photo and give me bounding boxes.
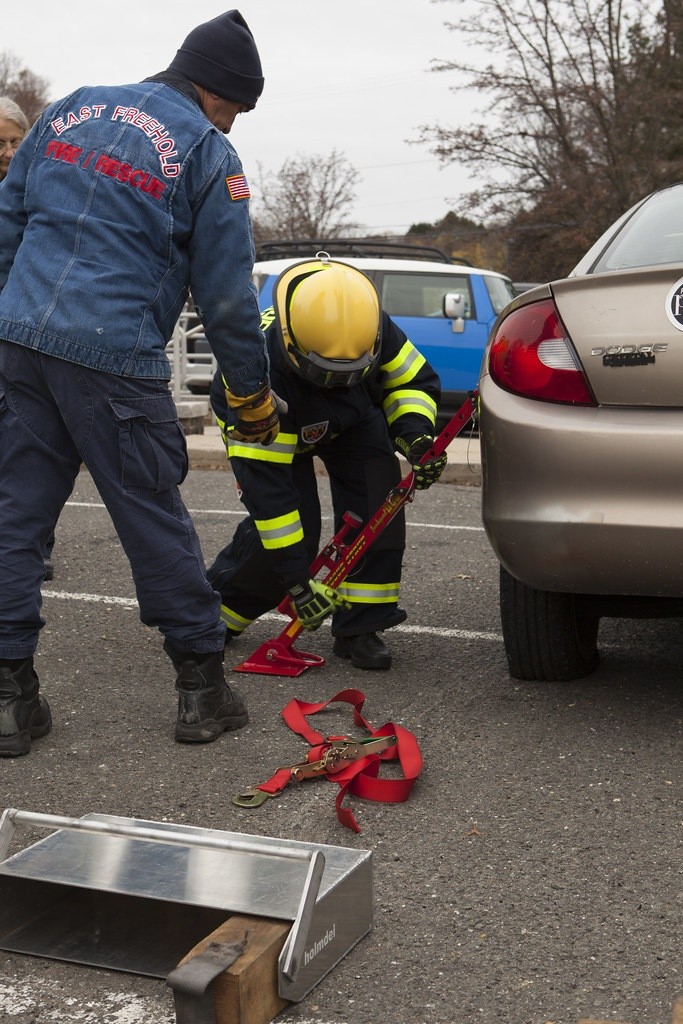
[284,569,352,631]
[394,431,448,490]
[223,385,288,446]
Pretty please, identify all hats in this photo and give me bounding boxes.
[165,9,264,109]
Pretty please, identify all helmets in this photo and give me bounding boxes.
[271,251,384,389]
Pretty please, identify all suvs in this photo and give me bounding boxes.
[196,232,523,436]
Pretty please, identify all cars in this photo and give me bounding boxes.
[478,181,683,684]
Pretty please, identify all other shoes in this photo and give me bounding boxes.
[333,633,392,670]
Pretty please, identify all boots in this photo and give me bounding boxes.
[168,647,249,743]
[0,656,53,757]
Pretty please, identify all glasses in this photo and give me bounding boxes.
[283,271,382,387]
[0,141,22,155]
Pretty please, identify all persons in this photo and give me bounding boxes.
[204,251,448,670]
[0,98,30,180]
[0,11,287,759]
